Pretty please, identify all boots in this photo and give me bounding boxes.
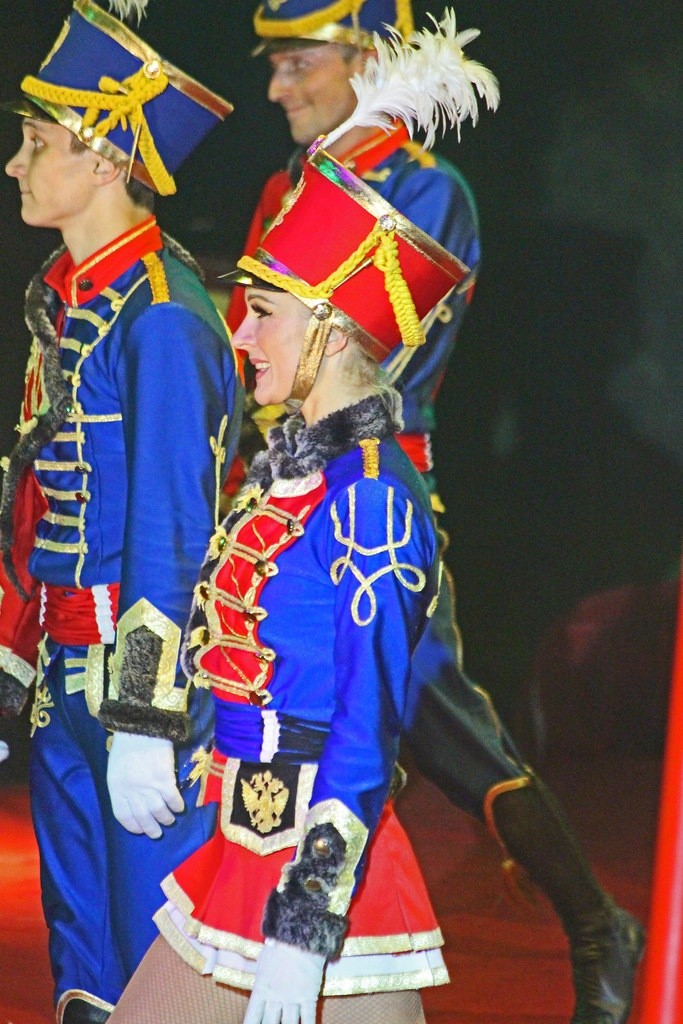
[483,764,645,1024]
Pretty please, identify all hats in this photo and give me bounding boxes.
[0,0,236,196]
[250,0,416,63]
[215,6,499,365]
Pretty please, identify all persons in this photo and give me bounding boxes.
[2,4,244,1024]
[113,150,474,1024]
[226,2,642,1024]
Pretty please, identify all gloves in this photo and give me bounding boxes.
[242,937,326,1024]
[106,730,185,840]
